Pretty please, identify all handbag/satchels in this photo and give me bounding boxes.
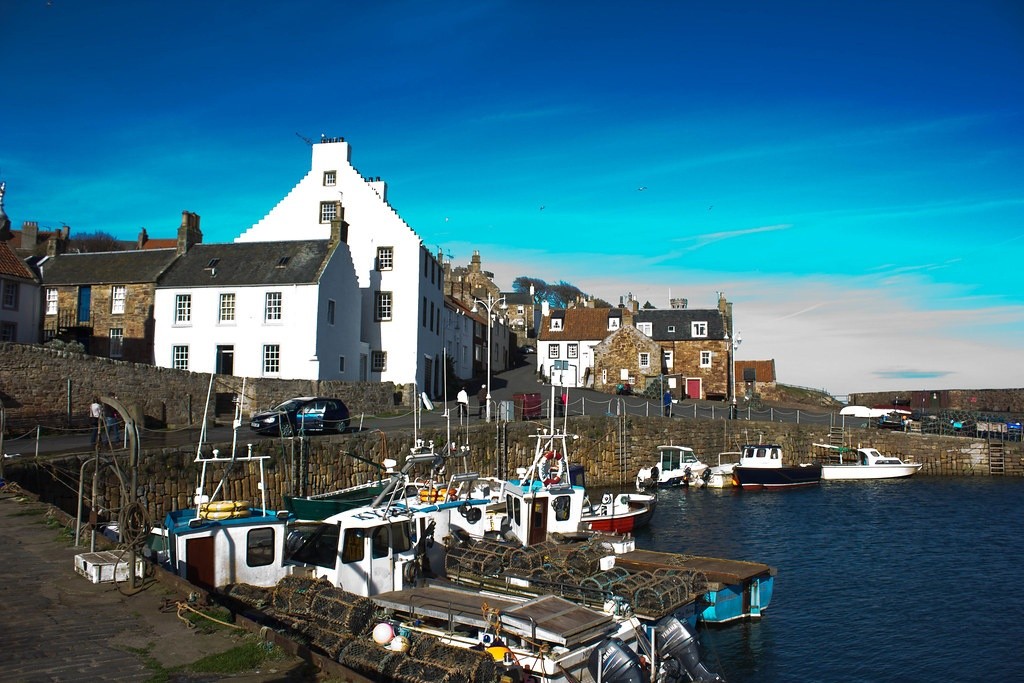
[89,417,99,426]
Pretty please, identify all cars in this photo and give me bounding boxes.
[519,345,537,354]
[879,413,905,431]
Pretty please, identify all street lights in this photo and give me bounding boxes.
[470,292,509,424]
[724,328,742,409]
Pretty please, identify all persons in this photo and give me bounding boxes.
[104,391,123,446]
[89,396,104,451]
[456,386,469,417]
[663,389,673,417]
[477,384,489,419]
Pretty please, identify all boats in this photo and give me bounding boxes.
[821,448,922,480]
[101,386,777,683]
[733,444,822,490]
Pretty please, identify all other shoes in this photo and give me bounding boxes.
[117,439,121,442]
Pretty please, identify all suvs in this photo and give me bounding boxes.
[249,396,350,437]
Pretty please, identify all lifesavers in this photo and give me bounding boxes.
[538,450,565,485]
[419,489,458,501]
[198,499,251,520]
